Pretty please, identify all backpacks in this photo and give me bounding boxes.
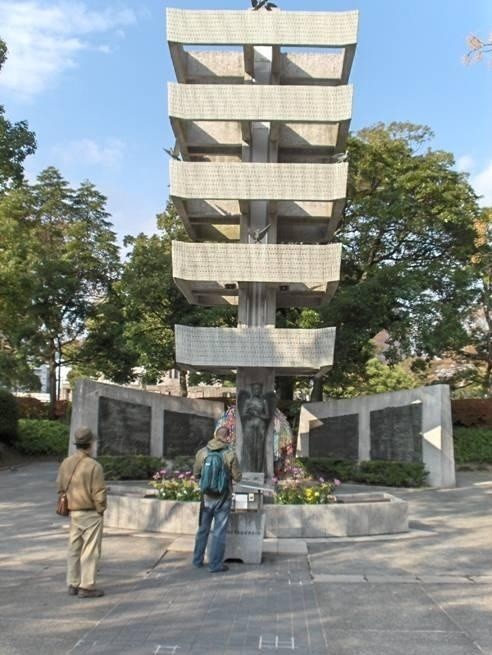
[201,447,231,495]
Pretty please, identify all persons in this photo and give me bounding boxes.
[57,428,107,599]
[192,426,242,572]
[243,382,272,473]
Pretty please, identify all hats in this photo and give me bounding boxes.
[72,427,94,444]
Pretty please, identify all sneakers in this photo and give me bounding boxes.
[209,565,229,572]
[68,587,104,598]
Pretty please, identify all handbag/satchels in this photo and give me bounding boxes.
[56,495,68,517]
